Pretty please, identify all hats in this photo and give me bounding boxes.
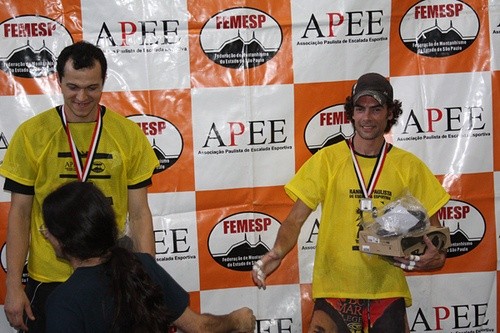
[351,73,394,105]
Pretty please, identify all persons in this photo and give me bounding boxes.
[33,180,257,332]
[308,295,349,332]
[252,73,451,333]
[0,37,161,333]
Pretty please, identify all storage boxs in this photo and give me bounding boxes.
[358,226,453,260]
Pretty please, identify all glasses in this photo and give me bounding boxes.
[38,223,50,235]
